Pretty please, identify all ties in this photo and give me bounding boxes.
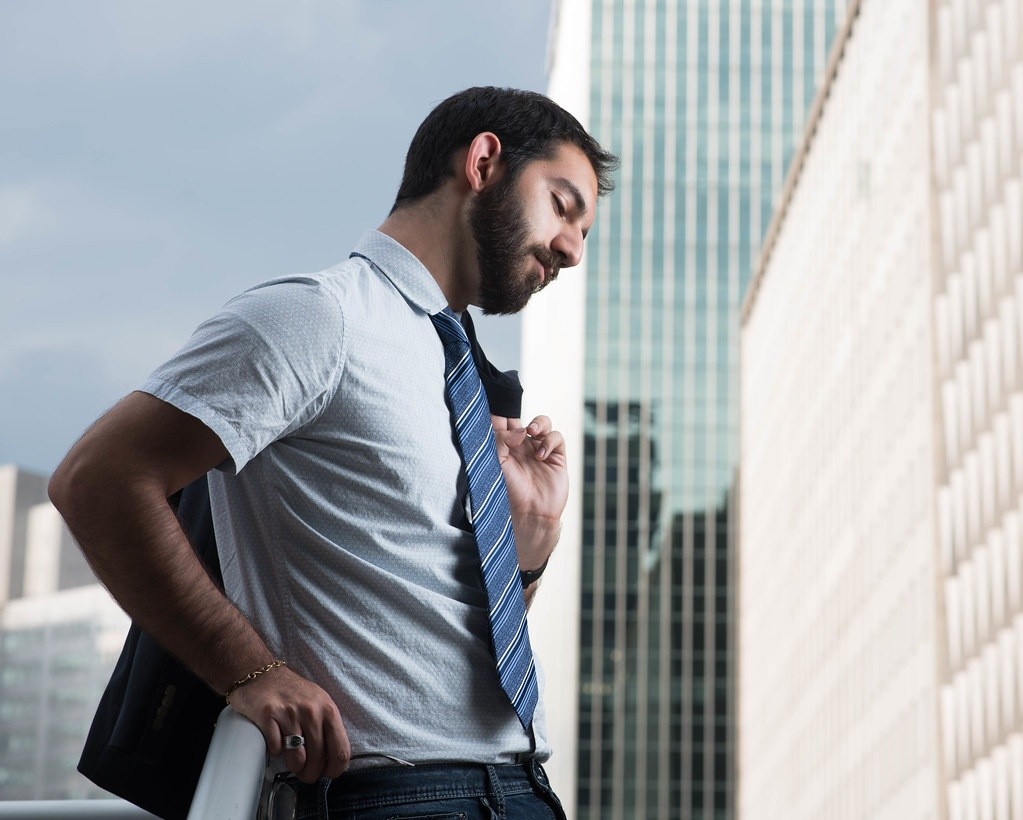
[428,305,540,735]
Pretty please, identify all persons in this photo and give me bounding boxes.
[46,85,619,820]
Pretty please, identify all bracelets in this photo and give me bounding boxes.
[221,659,287,704]
[519,555,549,589]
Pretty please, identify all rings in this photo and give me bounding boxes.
[282,735,305,750]
